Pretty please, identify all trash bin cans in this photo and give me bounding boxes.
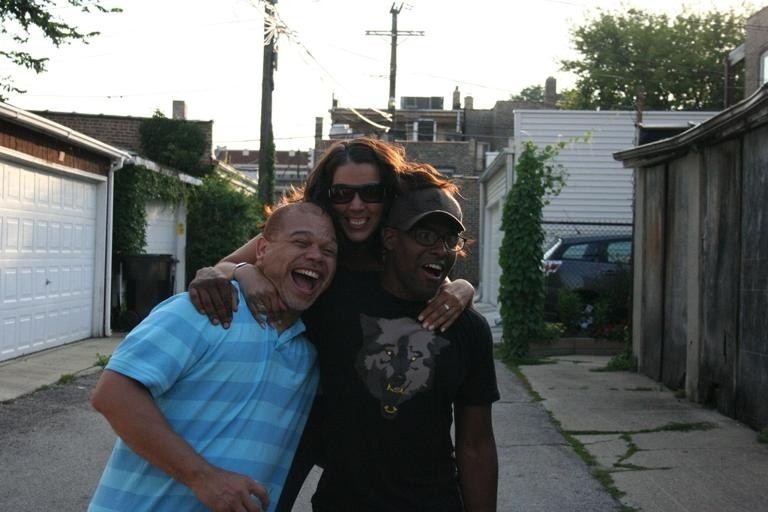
[119,254,180,322]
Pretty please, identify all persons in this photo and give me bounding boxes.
[189,184,502,510]
[82,199,342,511]
[212,136,476,510]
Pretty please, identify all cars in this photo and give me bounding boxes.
[541,206,635,339]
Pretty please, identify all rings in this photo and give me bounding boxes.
[443,302,451,311]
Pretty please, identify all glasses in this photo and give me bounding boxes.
[324,180,390,207]
[407,227,468,254]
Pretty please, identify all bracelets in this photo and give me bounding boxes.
[231,260,248,282]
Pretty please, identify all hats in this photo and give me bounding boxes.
[383,181,469,236]
